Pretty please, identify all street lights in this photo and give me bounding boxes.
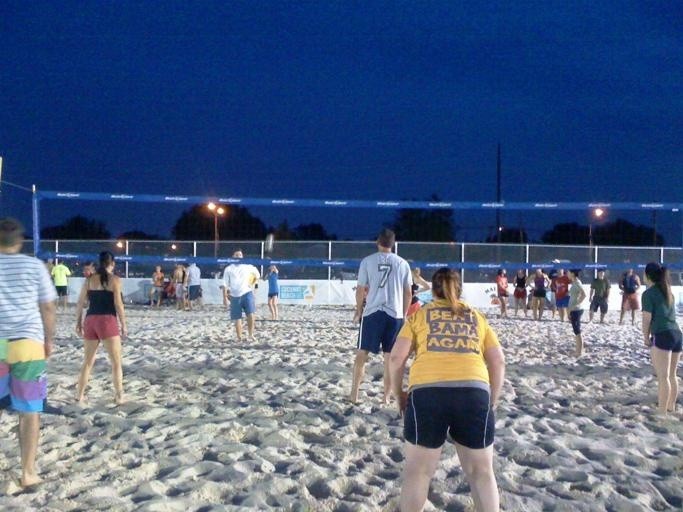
[206,201,226,258]
[587,208,604,263]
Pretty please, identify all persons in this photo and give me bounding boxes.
[44,258,72,310]
[150,260,202,311]
[388,267,505,512]
[223,250,260,343]
[0,218,58,487]
[642,261,683,418]
[496,258,655,357]
[83,260,95,278]
[75,250,129,405]
[262,263,281,319]
[407,268,430,316]
[349,229,414,406]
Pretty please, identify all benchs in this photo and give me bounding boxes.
[144,283,203,307]
